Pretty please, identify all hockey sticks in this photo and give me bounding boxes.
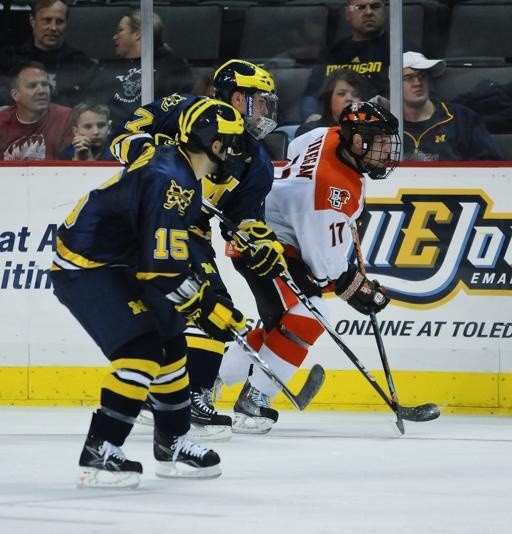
[350,225,404,435]
[201,196,440,421]
[228,325,325,411]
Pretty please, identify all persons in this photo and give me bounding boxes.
[104,59,289,441]
[225,99,401,436]
[1,0,90,108]
[300,0,408,126]
[90,8,192,128]
[57,100,125,161]
[0,62,76,160]
[388,51,504,161]
[293,68,372,138]
[48,94,246,489]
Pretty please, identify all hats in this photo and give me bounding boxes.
[402,51,446,78]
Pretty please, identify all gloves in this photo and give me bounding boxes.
[335,264,390,314]
[175,281,246,336]
[230,219,288,279]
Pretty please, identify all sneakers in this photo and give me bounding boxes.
[79,439,142,472]
[154,433,220,468]
[190,388,231,425]
[234,384,278,422]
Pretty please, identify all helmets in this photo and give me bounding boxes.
[213,60,279,140]
[179,98,244,184]
[339,102,401,179]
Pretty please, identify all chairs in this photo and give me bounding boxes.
[263,132,286,161]
[446,3,512,58]
[153,4,223,58]
[334,4,425,57]
[491,135,512,161]
[190,66,311,126]
[238,5,329,60]
[65,5,134,61]
[435,66,512,132]
